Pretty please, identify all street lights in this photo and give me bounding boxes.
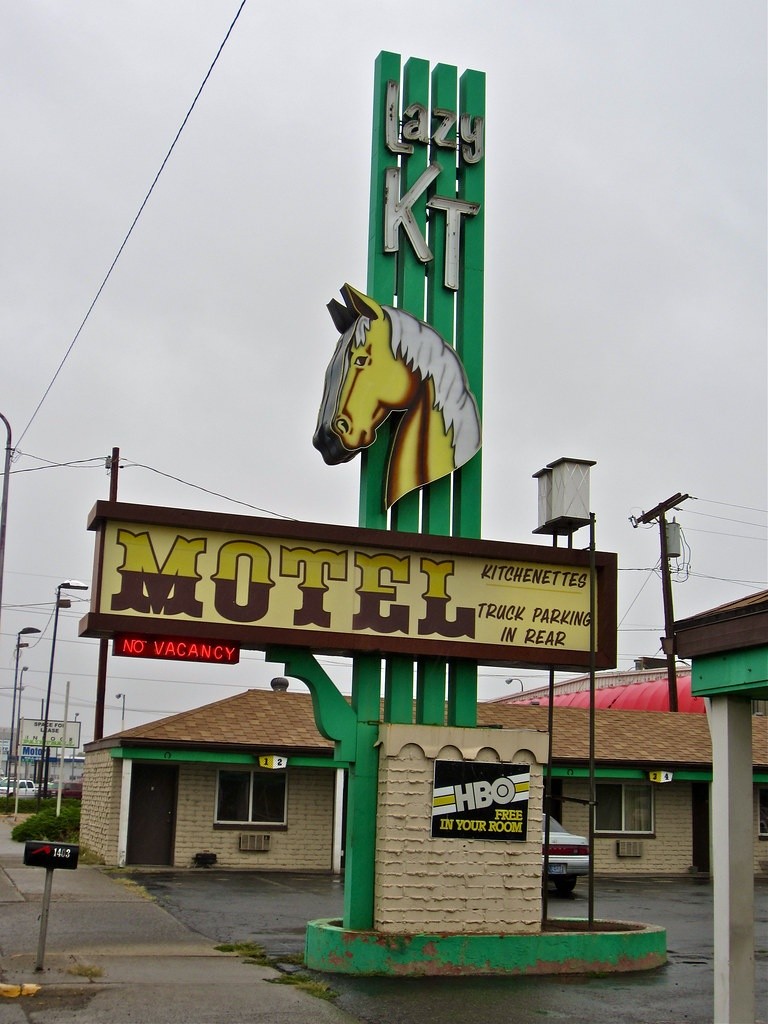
[13,666,31,798]
[71,712,80,780]
[115,693,126,732]
[34,582,90,813]
[5,627,45,810]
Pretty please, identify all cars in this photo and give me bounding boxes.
[0,779,37,799]
[40,780,82,800]
[540,813,589,894]
